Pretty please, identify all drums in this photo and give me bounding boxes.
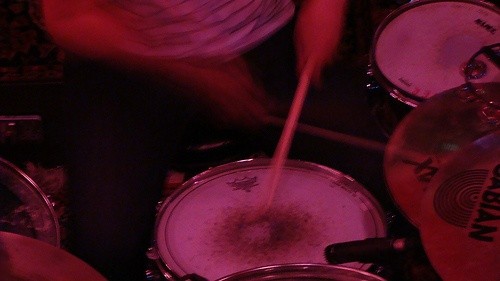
[138,155,393,281]
[214,261,385,281]
[0,110,79,250]
[363,0,499,138]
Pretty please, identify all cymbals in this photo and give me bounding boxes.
[384,83,500,243]
[418,227,500,281]
[1,231,105,281]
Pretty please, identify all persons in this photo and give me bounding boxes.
[39,0,349,281]
